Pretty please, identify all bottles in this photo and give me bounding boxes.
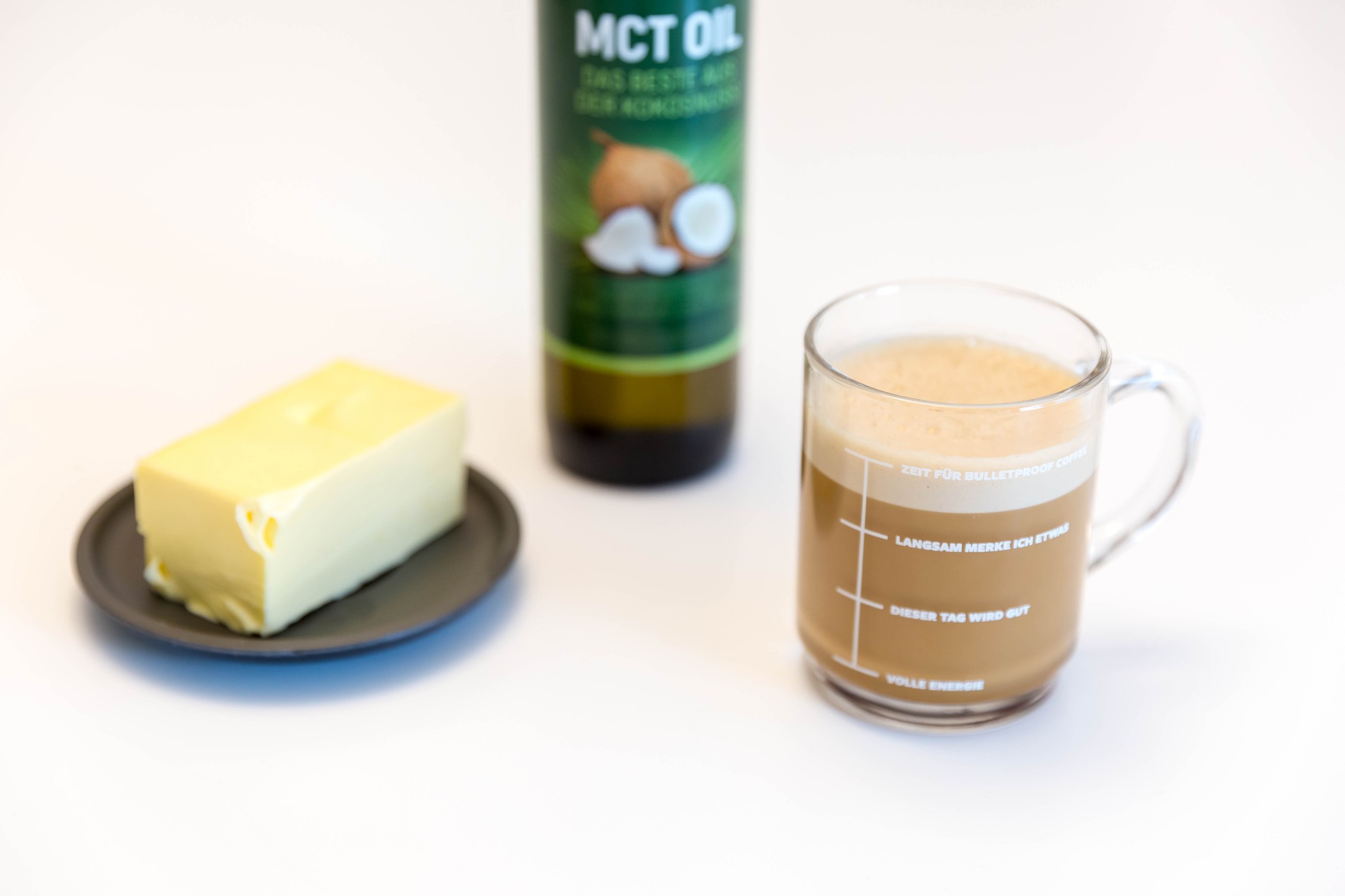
[533,2,754,492]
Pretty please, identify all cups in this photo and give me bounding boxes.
[795,276,1203,731]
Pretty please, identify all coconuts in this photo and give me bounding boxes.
[579,128,736,276]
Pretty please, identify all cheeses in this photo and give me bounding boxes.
[131,359,470,636]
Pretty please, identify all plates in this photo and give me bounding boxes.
[72,467,521,658]
